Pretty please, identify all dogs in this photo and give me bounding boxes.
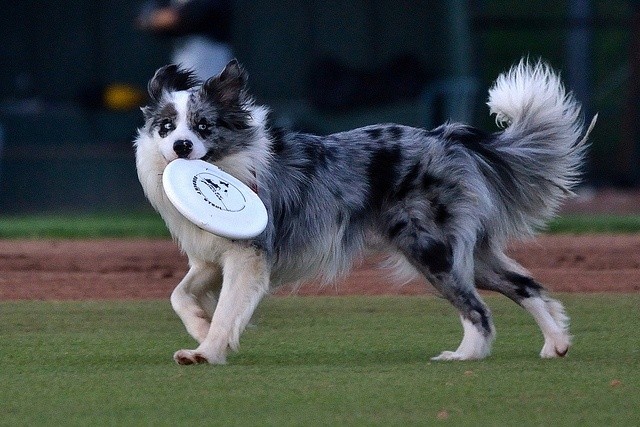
[132,57,598,367]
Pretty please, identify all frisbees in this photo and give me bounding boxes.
[161,158,269,238]
